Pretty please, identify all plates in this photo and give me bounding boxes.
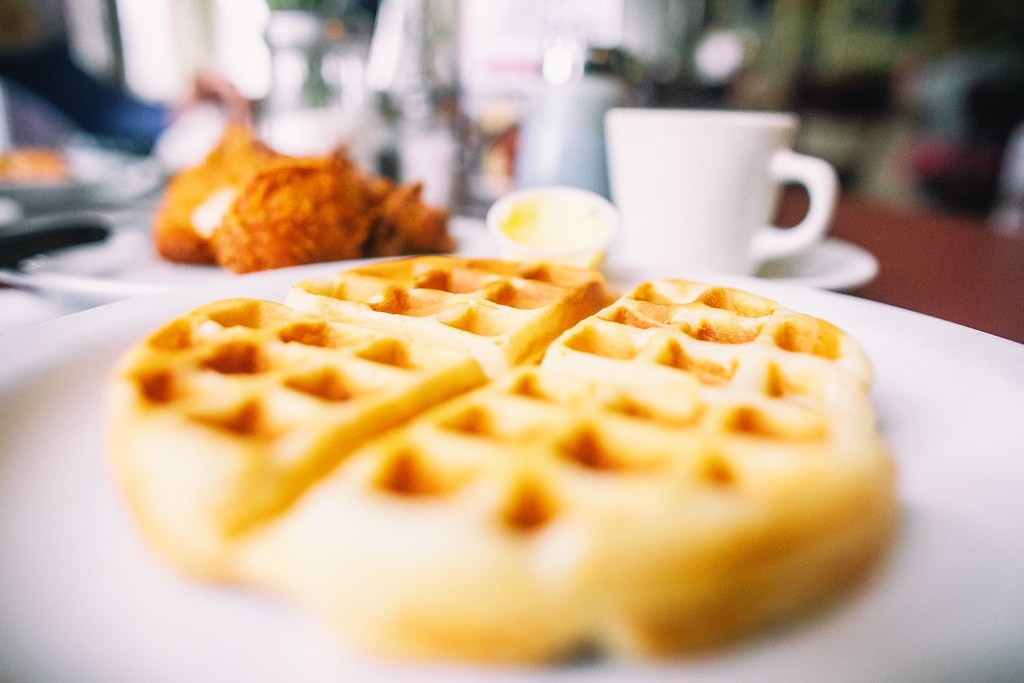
[748,238,880,290]
[0,264,1024,683]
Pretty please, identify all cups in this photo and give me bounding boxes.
[605,105,838,271]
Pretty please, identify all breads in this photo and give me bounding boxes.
[102,257,901,670]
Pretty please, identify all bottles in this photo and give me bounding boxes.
[256,12,343,157]
[392,83,461,216]
